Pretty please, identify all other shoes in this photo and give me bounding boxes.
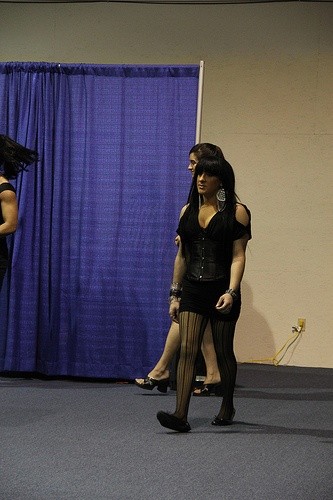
[211,407,237,426]
[157,411,191,433]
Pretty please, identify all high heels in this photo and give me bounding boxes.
[134,375,169,393]
[193,380,222,397]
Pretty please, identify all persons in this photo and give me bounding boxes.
[134,143,226,398]
[0,134,36,292]
[156,156,252,433]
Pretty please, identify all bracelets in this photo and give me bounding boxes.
[168,283,182,302]
[225,288,237,299]
